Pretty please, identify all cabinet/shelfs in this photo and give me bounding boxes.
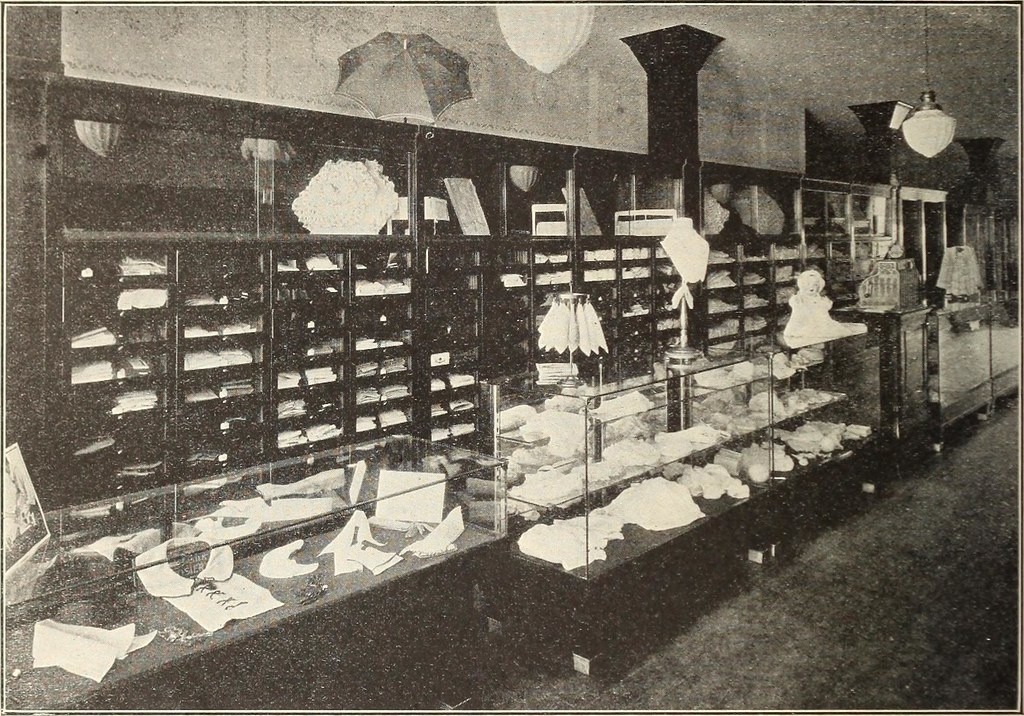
[6,178,903,712]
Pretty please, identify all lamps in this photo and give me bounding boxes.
[496,5,594,75]
[902,2,958,159]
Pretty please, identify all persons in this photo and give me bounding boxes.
[786,270,838,338]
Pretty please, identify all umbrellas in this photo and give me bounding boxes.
[335,32,475,123]
[734,190,785,234]
